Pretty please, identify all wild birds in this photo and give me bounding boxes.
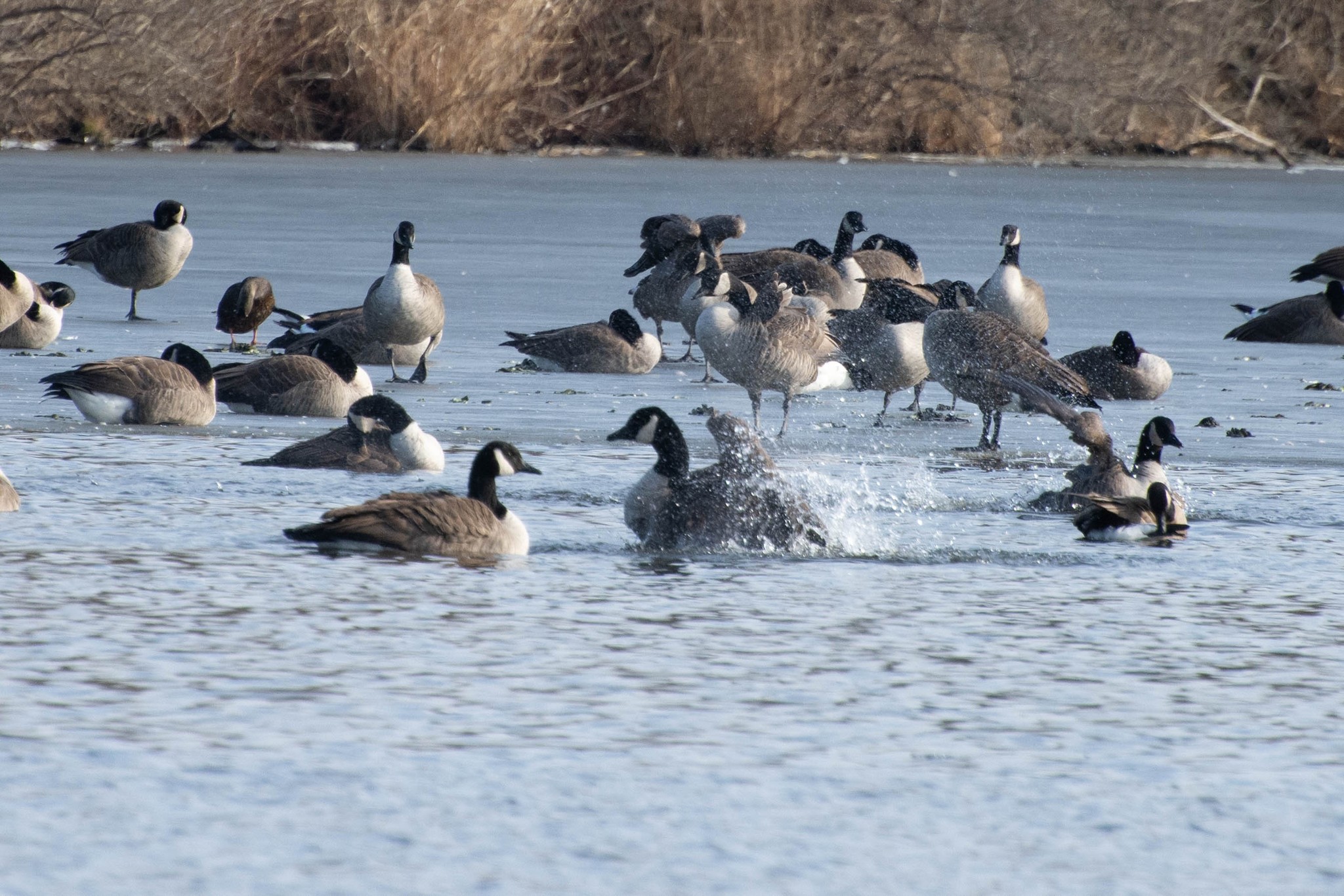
[501,212,1186,550]
[0,199,544,556]
[1223,246,1344,346]
